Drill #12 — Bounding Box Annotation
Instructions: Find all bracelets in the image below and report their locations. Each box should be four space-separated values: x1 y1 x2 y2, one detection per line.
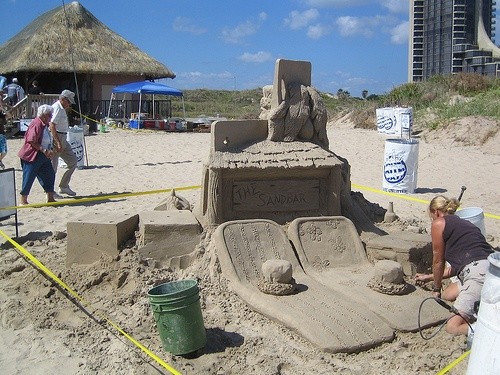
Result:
433 287 441 292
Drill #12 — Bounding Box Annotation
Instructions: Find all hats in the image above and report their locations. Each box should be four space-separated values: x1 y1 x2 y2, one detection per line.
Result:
61 89 76 105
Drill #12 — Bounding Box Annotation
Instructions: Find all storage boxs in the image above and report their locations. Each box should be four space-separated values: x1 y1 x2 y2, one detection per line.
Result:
130 119 144 129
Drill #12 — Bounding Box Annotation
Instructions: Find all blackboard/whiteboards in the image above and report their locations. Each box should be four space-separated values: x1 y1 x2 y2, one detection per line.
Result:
0 168 17 218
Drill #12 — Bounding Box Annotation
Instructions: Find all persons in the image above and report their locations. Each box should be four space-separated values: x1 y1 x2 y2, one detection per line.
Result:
415 195 495 334
28 80 44 119
18 104 63 208
0 109 8 169
43 89 78 199
3 78 21 121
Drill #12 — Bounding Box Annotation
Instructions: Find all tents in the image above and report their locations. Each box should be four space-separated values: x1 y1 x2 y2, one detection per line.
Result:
107 81 187 132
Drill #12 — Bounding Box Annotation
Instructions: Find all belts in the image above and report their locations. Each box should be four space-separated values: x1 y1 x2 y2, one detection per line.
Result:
56 131 67 134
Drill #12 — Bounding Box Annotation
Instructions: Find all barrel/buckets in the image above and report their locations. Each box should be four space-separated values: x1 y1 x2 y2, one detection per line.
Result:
454 207 486 239
382 138 420 195
147 280 207 355
58 126 85 171
466 251 500 375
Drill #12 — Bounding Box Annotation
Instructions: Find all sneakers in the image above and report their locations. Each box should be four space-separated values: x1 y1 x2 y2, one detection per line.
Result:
59 188 76 196
46 191 63 199
0 161 5 168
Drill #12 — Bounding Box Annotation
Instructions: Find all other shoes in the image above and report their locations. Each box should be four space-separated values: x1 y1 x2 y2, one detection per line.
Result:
18 203 36 208
46 201 61 206
